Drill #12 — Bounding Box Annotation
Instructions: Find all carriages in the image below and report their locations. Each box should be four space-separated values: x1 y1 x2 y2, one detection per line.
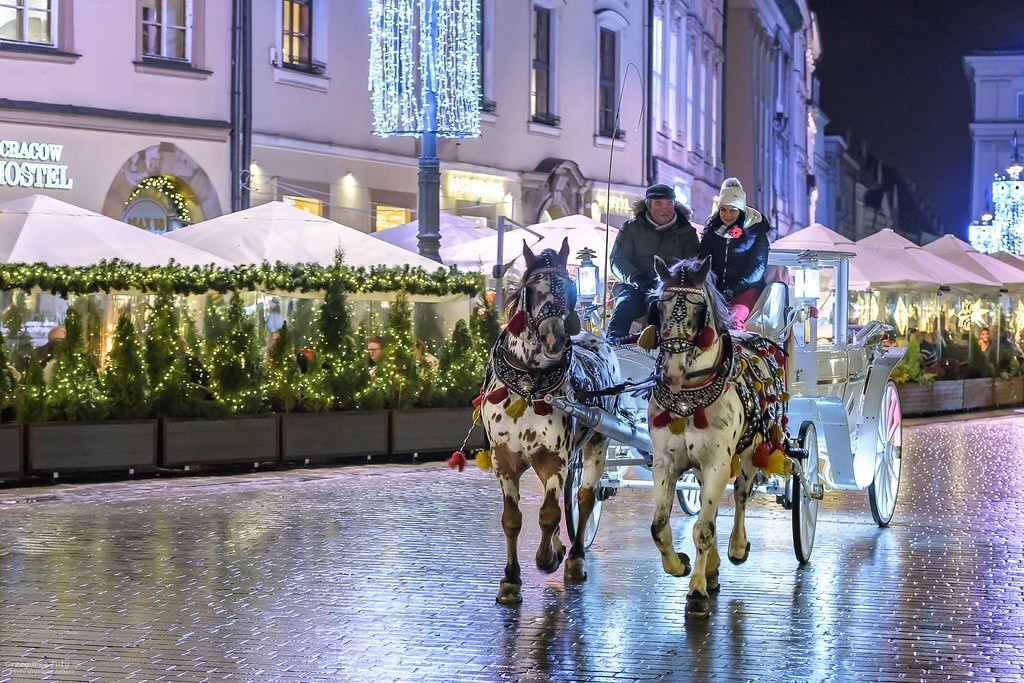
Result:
478 235 908 618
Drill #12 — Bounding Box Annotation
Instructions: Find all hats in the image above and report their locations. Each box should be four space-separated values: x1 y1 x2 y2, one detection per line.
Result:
645 183 676 199
718 177 746 213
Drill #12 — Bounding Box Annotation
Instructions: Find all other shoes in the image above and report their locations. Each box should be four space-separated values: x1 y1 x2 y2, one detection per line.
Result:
637 325 658 349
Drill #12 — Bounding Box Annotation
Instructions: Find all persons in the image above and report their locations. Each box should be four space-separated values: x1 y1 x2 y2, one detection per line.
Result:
698 177 770 334
605 183 701 351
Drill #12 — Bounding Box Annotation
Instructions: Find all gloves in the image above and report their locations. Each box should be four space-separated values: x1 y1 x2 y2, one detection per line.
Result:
721 286 734 300
631 274 654 293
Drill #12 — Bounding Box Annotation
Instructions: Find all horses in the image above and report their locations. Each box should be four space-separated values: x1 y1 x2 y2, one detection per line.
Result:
647 255 792 605
482 237 621 605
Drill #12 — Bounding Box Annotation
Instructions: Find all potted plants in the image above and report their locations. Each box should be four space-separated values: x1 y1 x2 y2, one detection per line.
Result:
891 332 1024 415
1 250 502 486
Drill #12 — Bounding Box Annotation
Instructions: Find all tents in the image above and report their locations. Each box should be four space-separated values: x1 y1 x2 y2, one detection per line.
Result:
3 195 1024 344
